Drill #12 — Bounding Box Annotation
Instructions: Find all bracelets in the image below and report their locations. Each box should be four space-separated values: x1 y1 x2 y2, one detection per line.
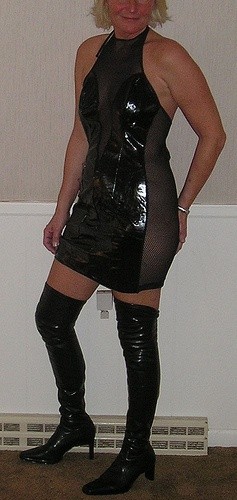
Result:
176 205 191 215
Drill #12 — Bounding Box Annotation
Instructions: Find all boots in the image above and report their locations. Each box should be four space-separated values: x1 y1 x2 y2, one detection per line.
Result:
17 282 97 463
82 296 161 496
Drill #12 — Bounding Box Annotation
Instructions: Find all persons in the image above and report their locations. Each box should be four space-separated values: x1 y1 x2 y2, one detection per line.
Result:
18 0 225 494
